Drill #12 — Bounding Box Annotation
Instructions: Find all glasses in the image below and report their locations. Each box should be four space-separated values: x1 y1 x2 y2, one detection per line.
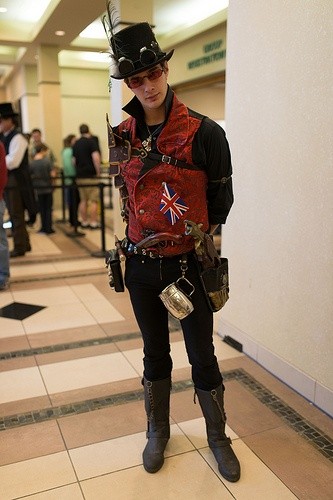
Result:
118 46 157 75
124 63 165 89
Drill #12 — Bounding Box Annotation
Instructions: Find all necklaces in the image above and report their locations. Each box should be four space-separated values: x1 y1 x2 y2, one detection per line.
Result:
143 122 165 151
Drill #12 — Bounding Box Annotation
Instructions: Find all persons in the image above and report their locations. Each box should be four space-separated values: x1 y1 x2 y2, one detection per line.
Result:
105 22 241 483
0 103 31 258
0 141 11 291
72 124 102 229
0 128 55 237
61 134 83 226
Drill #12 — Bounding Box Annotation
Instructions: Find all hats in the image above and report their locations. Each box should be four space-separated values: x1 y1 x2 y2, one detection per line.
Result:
100 0 175 79
0 103 19 119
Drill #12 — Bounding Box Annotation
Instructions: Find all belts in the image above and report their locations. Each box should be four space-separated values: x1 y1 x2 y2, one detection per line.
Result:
125 242 163 259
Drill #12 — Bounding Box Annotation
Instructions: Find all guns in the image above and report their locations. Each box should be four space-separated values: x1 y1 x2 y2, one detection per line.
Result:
184 219 221 271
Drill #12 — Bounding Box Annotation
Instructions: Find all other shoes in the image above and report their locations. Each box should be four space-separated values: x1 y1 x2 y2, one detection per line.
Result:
0 278 10 290
9 250 24 256
24 245 31 251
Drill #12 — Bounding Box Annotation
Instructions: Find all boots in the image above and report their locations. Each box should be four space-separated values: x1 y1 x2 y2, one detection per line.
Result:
142 373 171 474
193 378 240 483
